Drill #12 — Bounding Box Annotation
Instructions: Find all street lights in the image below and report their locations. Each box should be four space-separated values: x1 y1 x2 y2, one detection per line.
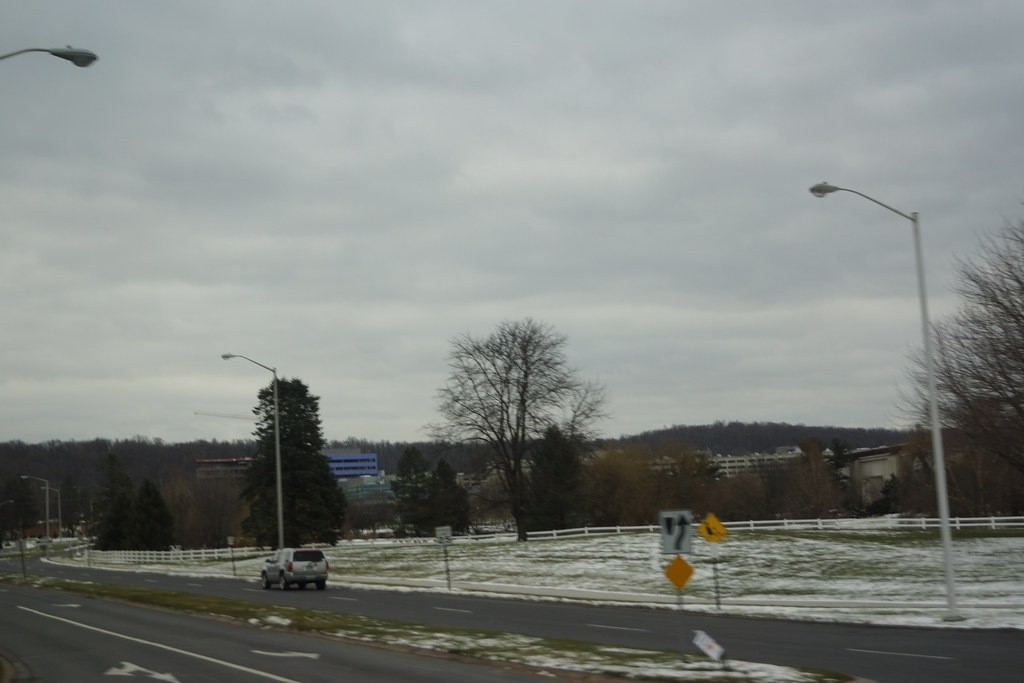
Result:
808 181 969 621
39 486 63 538
20 475 50 539
221 353 285 549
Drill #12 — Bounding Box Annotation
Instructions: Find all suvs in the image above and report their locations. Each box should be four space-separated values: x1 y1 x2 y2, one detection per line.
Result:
260 548 330 592
